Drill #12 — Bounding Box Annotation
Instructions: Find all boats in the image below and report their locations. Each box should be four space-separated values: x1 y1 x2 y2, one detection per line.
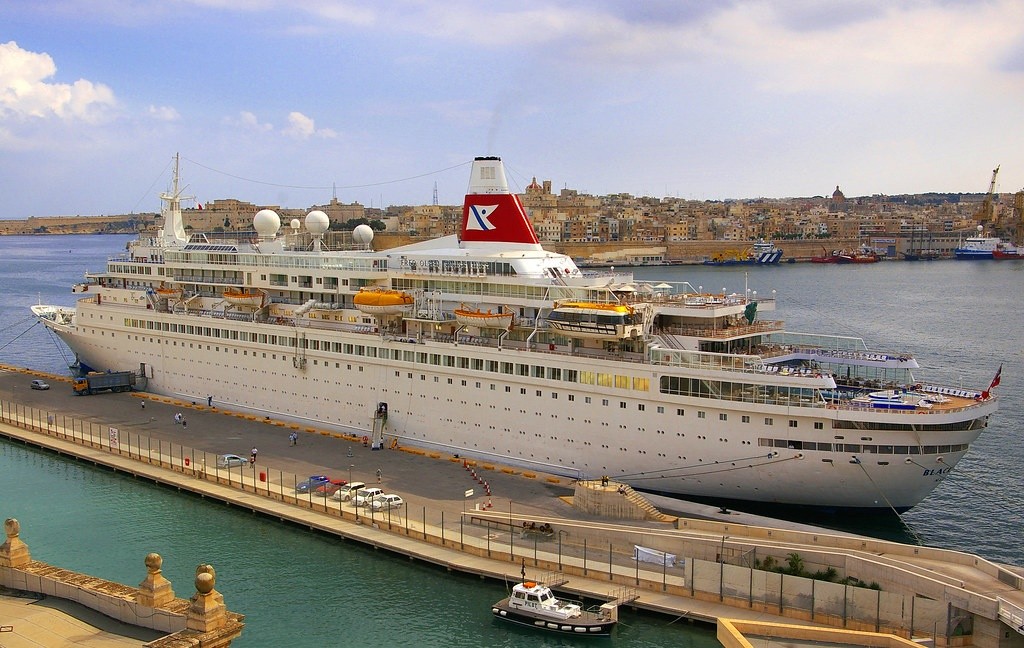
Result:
841 243 874 263
955 232 1017 259
155 288 182 298
545 302 644 339
634 544 676 564
353 291 414 313
704 237 784 265
453 309 512 328
491 557 617 637
223 291 267 304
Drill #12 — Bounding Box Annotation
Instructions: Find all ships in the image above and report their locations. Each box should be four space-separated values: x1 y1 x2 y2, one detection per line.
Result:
30 151 998 521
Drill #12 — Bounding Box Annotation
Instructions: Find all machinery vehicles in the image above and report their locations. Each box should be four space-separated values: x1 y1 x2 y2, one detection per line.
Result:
713 247 733 262
735 247 754 261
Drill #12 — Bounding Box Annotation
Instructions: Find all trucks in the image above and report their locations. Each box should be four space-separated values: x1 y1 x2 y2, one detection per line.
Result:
72 369 136 396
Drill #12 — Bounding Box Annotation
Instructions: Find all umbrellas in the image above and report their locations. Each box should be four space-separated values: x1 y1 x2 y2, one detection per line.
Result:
617 281 674 299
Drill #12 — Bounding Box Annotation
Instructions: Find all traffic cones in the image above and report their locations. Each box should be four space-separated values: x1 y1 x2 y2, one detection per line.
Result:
466 463 471 470
486 497 493 507
463 460 467 468
478 475 484 484
470 468 474 475
472 471 478 480
483 480 487 487
481 502 487 511
485 484 491 492
486 488 491 496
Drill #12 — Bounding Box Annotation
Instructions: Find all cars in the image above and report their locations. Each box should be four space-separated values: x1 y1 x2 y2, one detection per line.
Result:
368 494 403 511
296 476 330 493
334 482 366 501
217 454 247 468
352 488 384 507
316 479 347 496
31 380 49 389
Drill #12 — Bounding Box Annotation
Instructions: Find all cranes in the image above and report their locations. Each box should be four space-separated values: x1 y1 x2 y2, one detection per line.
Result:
973 164 1000 224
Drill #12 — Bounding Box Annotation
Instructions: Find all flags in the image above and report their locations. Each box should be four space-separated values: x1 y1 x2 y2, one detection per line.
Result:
198 204 202 210
991 365 1001 388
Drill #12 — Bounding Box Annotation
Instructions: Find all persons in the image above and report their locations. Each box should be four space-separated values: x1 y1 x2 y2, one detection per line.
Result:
618 485 627 495
289 432 297 446
379 437 383 449
249 455 254 468
174 410 186 430
376 469 382 484
207 396 212 406
141 400 145 408
601 474 608 486
378 404 386 418
252 447 257 461
